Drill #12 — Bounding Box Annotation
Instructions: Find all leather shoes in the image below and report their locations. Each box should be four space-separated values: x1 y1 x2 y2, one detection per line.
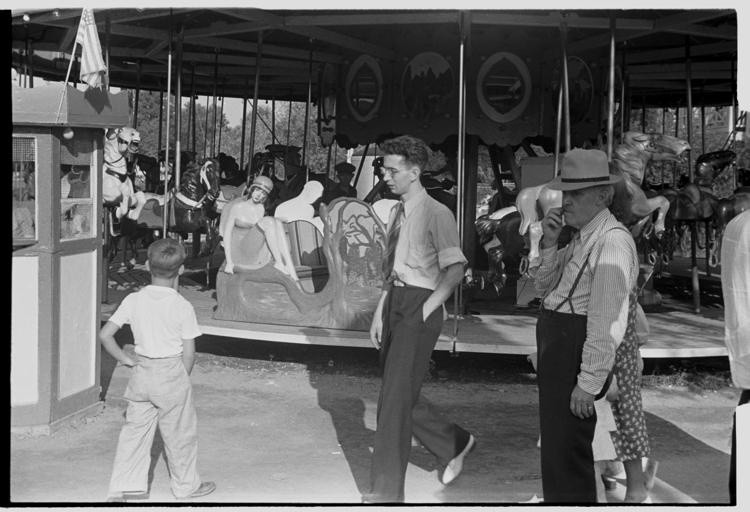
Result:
441 433 476 486
185 481 218 498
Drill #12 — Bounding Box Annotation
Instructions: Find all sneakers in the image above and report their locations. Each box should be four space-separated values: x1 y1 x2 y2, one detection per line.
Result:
642 458 660 491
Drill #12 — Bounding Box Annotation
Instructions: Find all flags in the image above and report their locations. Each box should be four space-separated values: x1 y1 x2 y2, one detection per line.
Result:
75 8 107 90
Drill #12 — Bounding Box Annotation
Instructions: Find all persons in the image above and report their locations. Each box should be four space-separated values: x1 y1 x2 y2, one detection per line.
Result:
364 135 475 503
605 285 659 505
97 237 216 504
529 148 641 502
224 159 400 281
719 209 749 505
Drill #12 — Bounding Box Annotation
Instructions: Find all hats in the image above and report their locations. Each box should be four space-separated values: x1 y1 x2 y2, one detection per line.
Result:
546 148 622 193
